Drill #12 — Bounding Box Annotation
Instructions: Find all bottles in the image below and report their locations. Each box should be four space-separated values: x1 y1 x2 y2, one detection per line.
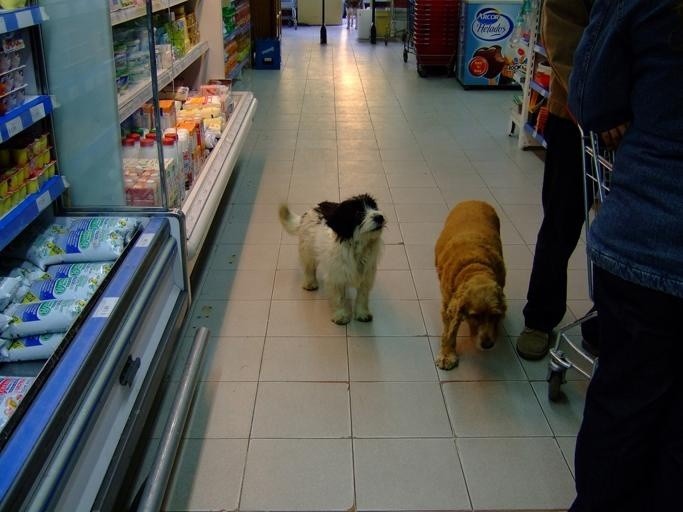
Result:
121 131 185 202
223 2 251 78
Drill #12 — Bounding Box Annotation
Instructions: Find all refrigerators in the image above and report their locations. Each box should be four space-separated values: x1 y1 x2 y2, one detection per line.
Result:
455 0 536 89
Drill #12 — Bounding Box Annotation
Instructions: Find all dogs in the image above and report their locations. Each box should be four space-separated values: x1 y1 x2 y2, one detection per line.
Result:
278 192 388 326
434 199 507 371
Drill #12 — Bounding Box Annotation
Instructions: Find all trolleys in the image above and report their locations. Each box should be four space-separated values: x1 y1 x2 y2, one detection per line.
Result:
547 123 614 401
384 7 413 62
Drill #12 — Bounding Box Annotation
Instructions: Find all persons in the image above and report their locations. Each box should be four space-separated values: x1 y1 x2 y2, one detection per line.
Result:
568 1 682 509
517 1 624 359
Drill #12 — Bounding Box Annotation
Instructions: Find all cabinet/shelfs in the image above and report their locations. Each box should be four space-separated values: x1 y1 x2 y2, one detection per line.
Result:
35 0 258 300
2 0 194 512
508 0 549 151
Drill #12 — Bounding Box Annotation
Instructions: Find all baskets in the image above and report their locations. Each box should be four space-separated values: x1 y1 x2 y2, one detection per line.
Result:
414 0 459 77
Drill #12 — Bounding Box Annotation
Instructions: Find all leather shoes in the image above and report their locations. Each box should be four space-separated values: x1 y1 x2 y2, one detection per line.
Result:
517 327 549 360
